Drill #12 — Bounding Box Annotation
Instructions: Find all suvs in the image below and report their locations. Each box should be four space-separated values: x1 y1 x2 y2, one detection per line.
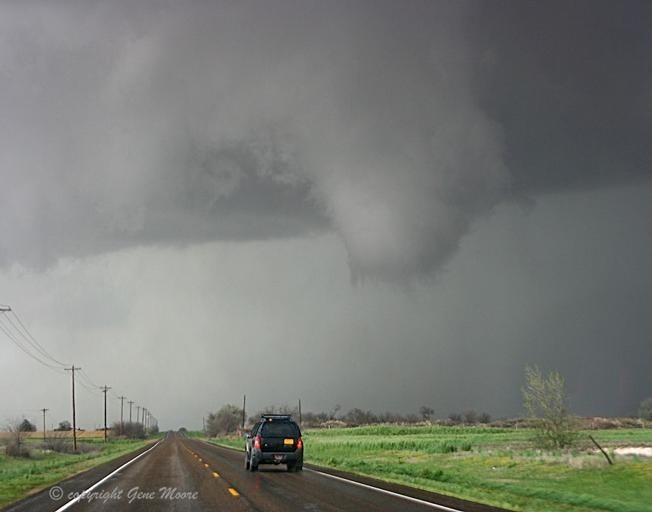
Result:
243 411 305 474
